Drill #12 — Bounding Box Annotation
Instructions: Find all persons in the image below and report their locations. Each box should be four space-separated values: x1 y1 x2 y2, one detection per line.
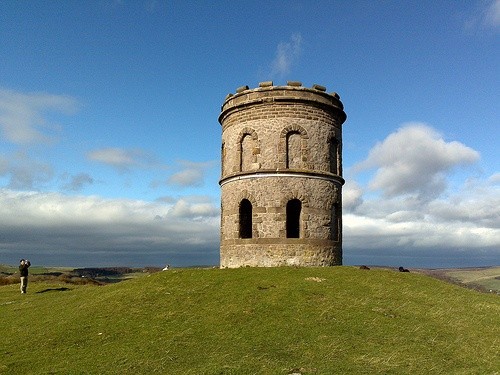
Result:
18 259 32 294
